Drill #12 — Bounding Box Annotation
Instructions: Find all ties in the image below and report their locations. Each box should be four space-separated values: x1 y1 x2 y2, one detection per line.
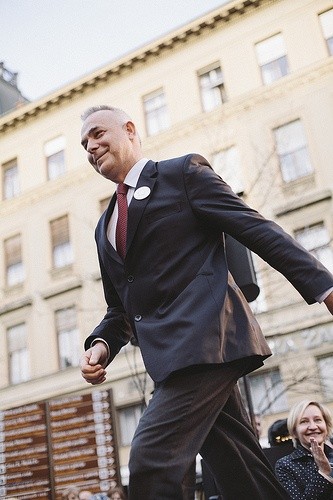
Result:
115 181 129 259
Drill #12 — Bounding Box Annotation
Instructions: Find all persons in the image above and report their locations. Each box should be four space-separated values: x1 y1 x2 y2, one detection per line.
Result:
248 399 333 500
78 105 332 500
